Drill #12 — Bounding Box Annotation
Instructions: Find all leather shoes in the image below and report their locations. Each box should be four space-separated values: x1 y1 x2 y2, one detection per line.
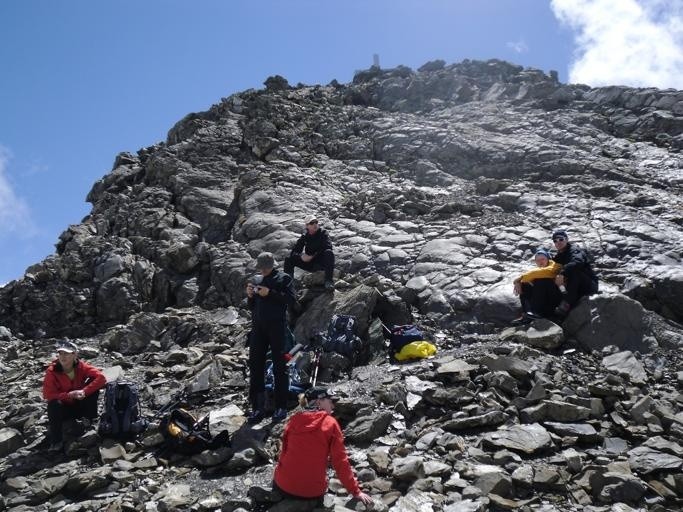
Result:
272 407 286 420
248 408 266 423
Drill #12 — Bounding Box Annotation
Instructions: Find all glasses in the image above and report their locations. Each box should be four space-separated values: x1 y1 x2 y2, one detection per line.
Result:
59 352 73 356
552 237 565 243
309 220 318 225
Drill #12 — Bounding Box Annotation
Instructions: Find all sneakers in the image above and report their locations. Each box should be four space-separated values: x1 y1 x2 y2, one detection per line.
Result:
48 442 64 453
221 498 255 512
325 282 335 292
509 300 570 326
248 485 282 503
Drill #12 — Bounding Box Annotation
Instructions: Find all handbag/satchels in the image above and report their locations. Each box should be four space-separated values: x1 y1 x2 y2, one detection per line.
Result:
160 408 230 454
389 324 425 353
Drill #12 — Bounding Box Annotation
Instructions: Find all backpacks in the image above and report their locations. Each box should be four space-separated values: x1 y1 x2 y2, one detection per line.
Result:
327 313 358 343
98 378 148 437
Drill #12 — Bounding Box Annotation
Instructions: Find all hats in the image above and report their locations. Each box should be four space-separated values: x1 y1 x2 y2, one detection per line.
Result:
57 342 80 353
536 249 549 258
553 230 567 240
304 387 340 403
254 252 279 270
305 214 317 224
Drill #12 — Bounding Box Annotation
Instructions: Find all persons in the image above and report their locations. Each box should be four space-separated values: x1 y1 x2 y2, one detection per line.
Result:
283 213 335 295
510 247 565 318
246 248 295 422
42 340 103 438
246 386 372 506
549 231 601 309
